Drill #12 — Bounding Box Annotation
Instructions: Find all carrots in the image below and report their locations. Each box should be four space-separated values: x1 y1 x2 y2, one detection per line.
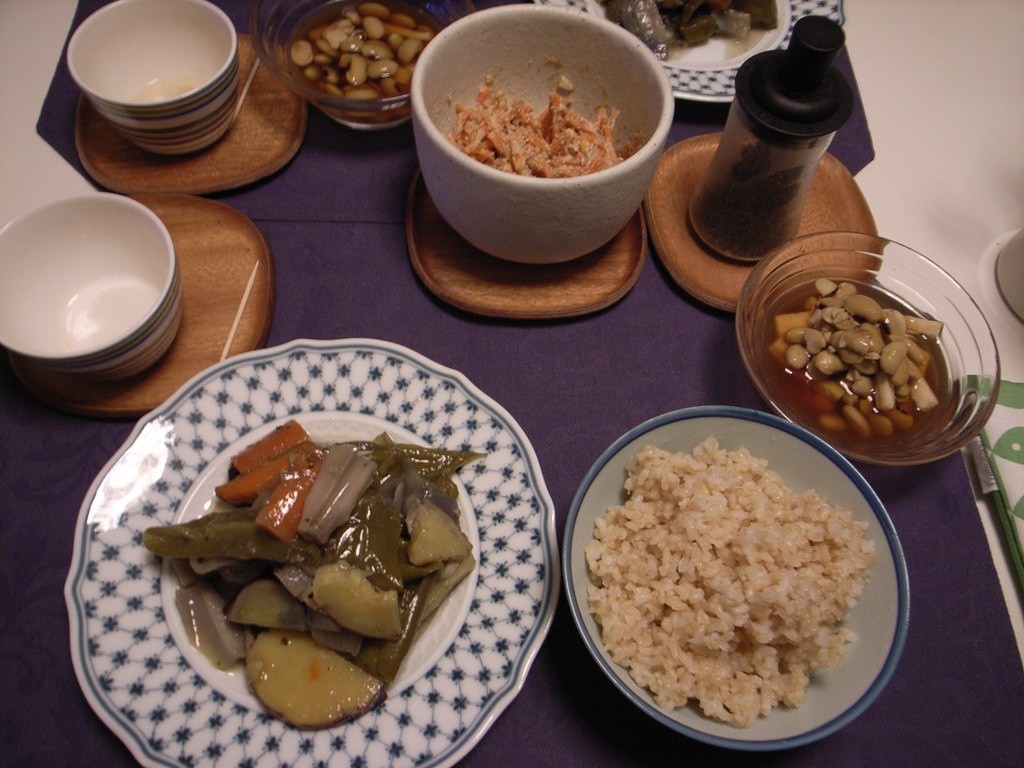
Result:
216 419 329 543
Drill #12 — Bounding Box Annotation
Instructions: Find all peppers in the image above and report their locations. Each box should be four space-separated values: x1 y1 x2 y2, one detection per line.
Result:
142 432 493 683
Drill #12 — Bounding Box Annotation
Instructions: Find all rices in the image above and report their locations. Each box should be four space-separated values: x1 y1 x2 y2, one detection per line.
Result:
584 434 875 729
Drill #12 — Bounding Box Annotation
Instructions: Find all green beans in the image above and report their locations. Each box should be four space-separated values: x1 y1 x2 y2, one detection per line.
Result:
304 2 436 99
601 1 777 45
781 278 926 440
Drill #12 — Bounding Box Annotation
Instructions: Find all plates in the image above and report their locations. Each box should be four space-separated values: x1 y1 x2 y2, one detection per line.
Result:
531 0 845 102
74 32 307 193
644 130 882 311
63 336 560 768
18 191 274 414
405 163 648 319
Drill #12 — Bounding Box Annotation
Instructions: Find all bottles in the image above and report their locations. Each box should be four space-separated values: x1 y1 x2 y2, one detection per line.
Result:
689 15 855 265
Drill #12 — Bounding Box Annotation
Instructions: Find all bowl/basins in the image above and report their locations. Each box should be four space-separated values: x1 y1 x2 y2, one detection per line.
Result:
735 230 1002 465
66 0 240 158
410 4 674 265
562 405 912 751
0 192 183 381
248 0 477 132
